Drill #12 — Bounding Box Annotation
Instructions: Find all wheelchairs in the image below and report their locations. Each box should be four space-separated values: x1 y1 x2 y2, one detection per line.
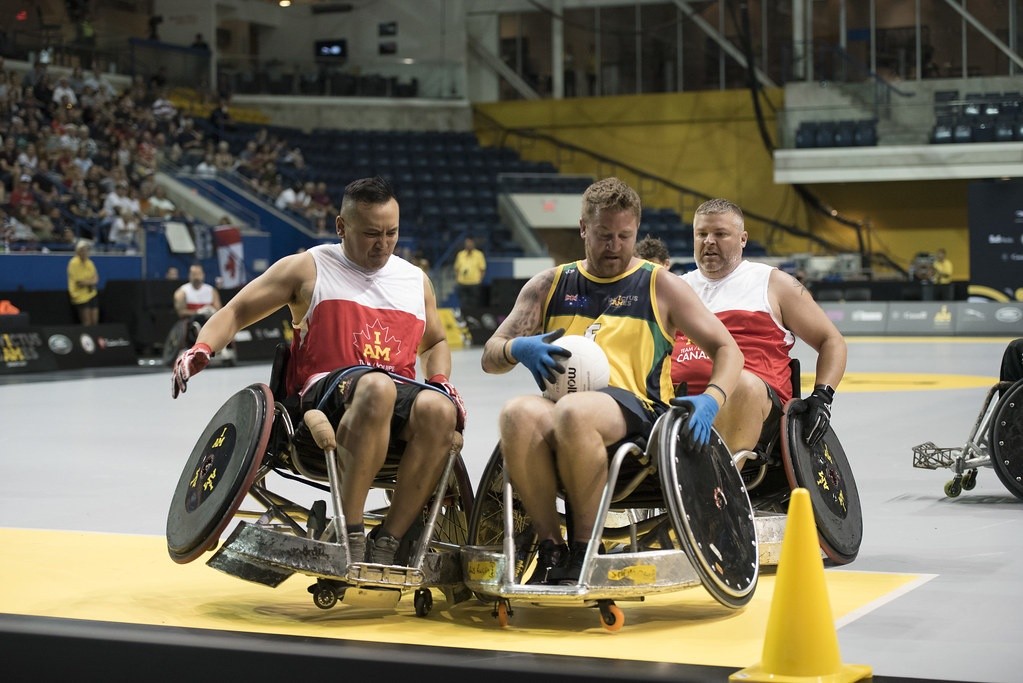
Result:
911 338 1023 503
165 342 476 618
626 359 864 566
462 381 760 631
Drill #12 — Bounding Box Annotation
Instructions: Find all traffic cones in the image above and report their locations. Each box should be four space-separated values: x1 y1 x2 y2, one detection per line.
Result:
727 486 874 682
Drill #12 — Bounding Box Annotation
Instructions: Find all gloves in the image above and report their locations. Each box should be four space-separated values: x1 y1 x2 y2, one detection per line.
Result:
668 394 719 453
170 343 211 400
427 374 465 432
512 327 572 391
792 383 835 448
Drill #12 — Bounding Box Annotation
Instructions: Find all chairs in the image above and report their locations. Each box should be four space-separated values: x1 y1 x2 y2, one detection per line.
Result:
208 125 697 272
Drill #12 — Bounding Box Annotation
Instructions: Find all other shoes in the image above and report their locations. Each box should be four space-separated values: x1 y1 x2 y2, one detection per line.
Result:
347 533 366 562
552 541 607 588
520 539 569 585
365 532 400 568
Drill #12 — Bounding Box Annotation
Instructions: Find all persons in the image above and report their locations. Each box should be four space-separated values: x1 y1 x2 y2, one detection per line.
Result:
170 178 464 565
0 32 433 359
999 339 1023 396
931 248 954 300
482 177 745 585
672 199 848 474
633 234 671 271
453 235 487 318
